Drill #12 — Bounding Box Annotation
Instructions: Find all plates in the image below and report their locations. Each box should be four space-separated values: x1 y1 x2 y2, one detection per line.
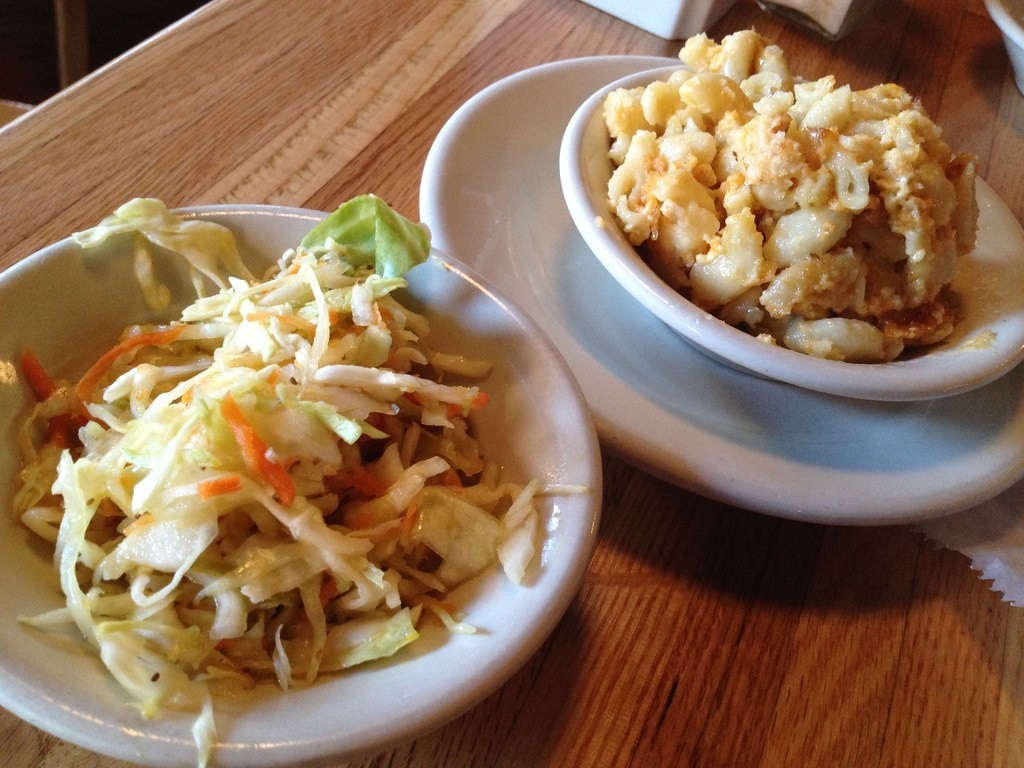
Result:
423 52 1024 522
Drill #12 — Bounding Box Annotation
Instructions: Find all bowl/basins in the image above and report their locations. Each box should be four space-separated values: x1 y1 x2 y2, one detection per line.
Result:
559 64 1024 405
0 201 604 768
983 2 1024 98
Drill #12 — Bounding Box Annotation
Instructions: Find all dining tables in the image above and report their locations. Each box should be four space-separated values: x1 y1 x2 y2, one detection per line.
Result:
0 0 1023 768
